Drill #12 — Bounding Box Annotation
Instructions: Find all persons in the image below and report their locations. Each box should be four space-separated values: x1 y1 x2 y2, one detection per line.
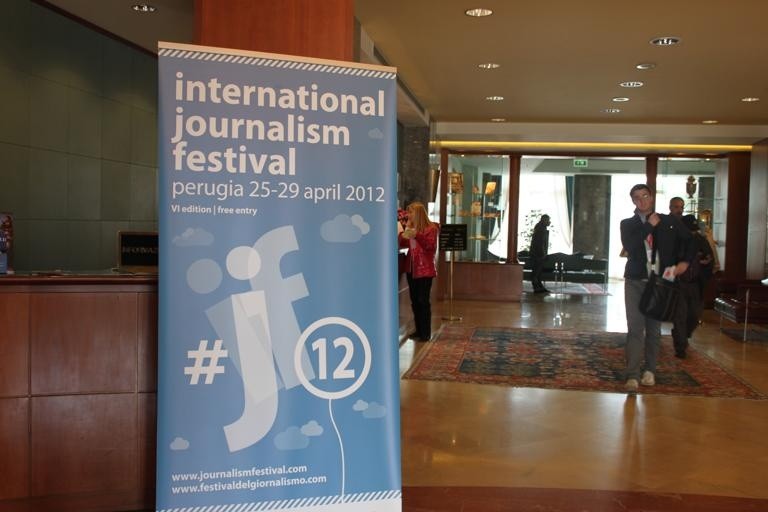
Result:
530 214 550 293
620 184 697 391
398 202 437 342
672 214 714 359
667 197 684 219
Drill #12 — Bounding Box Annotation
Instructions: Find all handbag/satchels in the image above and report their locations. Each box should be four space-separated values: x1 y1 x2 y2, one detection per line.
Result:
638 273 689 323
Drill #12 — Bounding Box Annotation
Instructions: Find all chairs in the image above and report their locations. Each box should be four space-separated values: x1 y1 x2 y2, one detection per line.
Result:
484 246 612 300
708 274 767 344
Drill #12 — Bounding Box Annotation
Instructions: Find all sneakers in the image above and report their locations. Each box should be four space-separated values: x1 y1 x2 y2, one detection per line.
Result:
672 328 688 358
624 377 639 392
534 287 551 293
408 330 430 342
640 371 655 385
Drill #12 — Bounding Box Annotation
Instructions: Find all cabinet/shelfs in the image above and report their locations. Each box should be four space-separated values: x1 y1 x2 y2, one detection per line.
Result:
682 195 727 250
444 191 507 248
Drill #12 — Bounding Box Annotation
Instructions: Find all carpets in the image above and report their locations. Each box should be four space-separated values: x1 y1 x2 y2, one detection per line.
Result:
399 321 767 403
